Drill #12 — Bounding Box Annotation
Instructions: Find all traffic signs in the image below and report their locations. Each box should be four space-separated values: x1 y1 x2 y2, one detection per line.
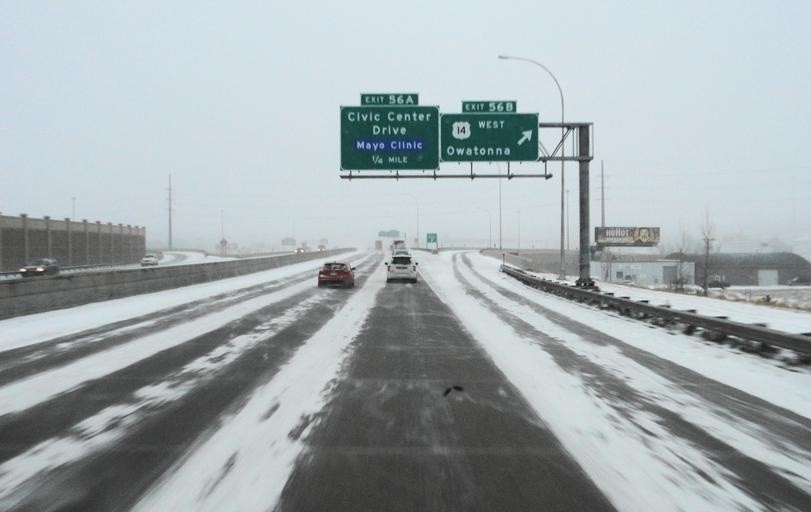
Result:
379 229 401 238
463 102 518 113
363 93 419 106
342 104 438 169
441 112 539 162
427 233 438 242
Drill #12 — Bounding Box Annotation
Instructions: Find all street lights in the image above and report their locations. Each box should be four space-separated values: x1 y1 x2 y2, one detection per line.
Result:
498 54 569 279
72 195 78 221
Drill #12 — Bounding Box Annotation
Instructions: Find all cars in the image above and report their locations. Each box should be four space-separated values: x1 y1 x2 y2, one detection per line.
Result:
142 253 159 265
702 280 729 288
318 245 327 251
788 276 810 286
672 277 690 285
295 247 305 255
386 239 418 282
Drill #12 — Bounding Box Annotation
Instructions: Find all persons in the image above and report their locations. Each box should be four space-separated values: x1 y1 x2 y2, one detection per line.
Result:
633 227 657 245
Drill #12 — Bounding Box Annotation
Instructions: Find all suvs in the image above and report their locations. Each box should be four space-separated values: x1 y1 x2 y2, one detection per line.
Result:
318 259 357 288
20 258 60 277
